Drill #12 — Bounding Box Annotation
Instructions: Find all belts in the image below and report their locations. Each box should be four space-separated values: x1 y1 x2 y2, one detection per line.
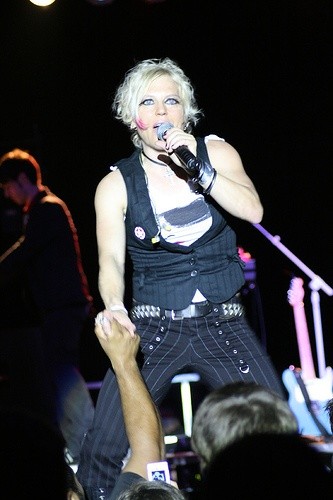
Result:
131 303 244 320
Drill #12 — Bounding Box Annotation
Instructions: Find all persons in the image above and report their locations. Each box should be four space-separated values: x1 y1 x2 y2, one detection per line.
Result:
0 309 333 500
76 56 286 500
0 148 96 405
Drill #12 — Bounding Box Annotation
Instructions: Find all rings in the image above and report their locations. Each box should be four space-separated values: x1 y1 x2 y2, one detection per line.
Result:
98 316 107 323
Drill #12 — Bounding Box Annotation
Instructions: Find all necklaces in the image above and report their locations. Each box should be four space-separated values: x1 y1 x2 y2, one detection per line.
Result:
139 148 177 176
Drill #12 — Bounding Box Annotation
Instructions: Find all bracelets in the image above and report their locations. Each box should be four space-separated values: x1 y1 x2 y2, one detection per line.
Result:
192 158 217 195
110 305 128 314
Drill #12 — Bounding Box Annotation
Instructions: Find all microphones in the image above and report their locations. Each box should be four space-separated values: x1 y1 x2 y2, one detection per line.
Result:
157 122 202 171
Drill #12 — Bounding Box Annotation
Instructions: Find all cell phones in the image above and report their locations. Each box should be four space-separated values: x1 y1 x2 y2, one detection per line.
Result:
147 461 170 484
